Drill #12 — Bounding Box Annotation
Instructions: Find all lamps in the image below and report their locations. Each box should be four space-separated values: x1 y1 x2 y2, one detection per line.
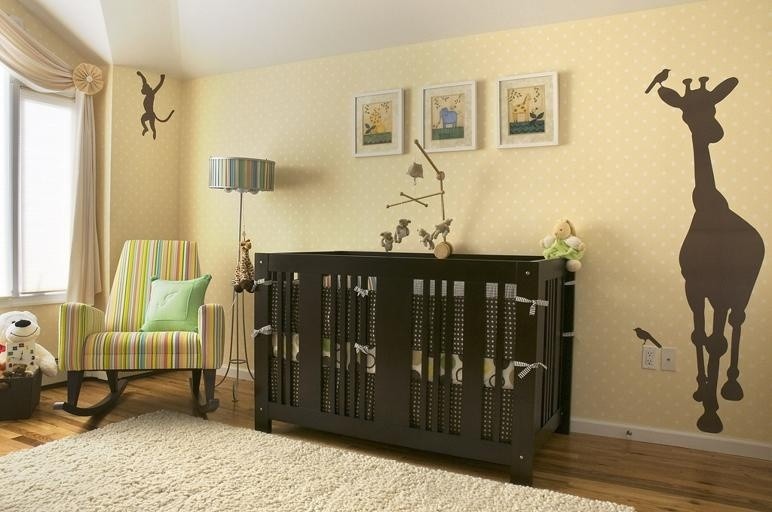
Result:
207 156 276 401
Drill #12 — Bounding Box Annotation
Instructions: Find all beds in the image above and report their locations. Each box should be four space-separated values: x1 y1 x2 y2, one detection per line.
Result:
249 248 579 488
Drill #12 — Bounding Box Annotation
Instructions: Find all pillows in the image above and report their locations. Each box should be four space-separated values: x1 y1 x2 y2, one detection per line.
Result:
135 271 214 332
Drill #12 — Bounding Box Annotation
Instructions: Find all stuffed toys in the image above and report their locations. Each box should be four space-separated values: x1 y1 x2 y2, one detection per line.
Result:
0 311 58 388
539 222 585 272
233 238 255 293
380 138 454 259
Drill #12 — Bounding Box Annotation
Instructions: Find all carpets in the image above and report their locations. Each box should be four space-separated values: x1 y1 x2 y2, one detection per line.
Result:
2 406 639 511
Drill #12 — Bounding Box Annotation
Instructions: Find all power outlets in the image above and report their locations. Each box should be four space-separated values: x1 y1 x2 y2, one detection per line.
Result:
641 345 658 370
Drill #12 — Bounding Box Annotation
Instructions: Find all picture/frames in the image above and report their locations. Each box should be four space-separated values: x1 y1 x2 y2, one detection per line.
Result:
352 87 406 159
497 71 560 150
420 81 478 153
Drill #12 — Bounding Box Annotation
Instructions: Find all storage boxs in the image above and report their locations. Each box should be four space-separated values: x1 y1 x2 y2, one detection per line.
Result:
0 373 43 422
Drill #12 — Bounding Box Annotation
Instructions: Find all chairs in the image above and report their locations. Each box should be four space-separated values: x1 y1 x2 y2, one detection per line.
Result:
53 234 227 420
